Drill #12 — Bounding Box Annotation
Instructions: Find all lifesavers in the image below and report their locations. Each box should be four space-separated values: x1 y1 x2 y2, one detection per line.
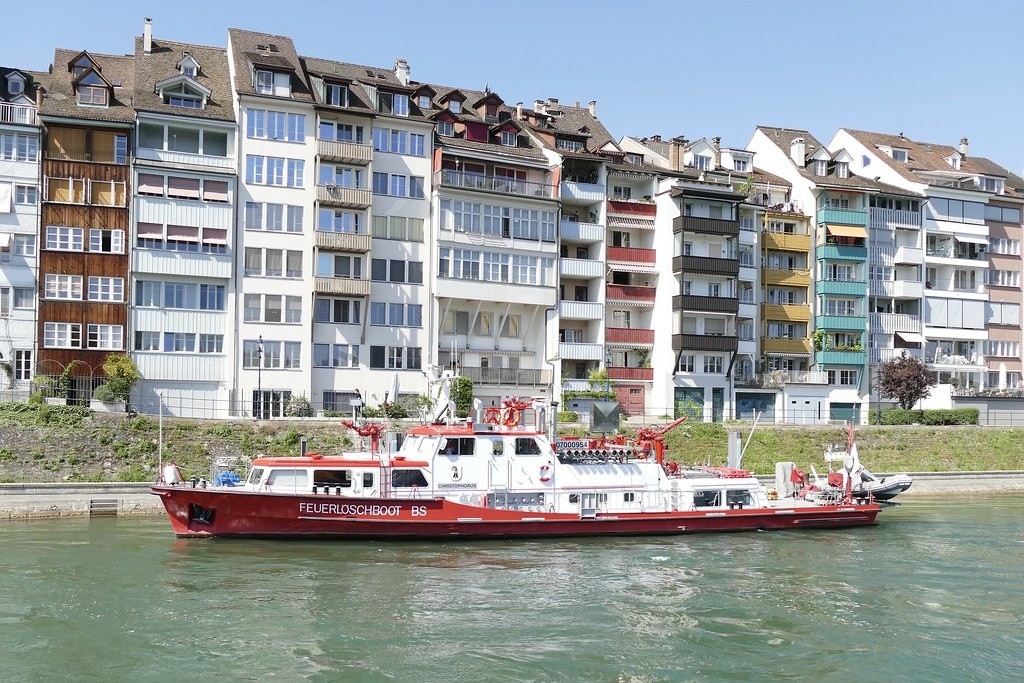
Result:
501 406 522 428
632 440 651 460
483 406 502 426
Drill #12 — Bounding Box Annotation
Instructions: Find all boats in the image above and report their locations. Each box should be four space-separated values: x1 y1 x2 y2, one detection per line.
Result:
810 400 913 501
148 396 882 542
